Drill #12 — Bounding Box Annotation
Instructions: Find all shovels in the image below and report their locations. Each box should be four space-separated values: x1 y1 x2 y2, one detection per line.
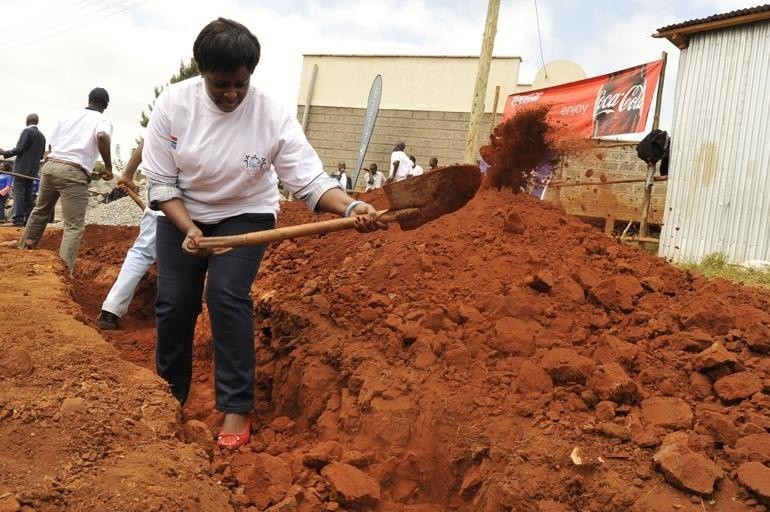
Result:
188 164 481 250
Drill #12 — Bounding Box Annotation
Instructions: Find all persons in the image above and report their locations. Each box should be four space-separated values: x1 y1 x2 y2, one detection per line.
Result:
329 141 438 196
142 17 389 448
17 88 112 279
95 136 157 329
0 114 46 226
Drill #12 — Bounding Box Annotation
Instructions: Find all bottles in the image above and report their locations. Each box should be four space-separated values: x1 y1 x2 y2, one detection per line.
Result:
590 70 624 138
624 64 649 134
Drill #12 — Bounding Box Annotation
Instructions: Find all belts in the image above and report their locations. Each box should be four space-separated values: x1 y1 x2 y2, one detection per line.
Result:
47 157 87 175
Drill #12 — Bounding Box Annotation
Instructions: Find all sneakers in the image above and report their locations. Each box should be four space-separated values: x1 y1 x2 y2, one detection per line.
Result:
95 310 118 329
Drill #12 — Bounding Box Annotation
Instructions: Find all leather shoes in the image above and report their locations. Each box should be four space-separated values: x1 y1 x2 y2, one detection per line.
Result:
12 216 24 225
217 415 251 449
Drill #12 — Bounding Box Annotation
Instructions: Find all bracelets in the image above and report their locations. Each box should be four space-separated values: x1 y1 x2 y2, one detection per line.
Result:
345 201 362 217
